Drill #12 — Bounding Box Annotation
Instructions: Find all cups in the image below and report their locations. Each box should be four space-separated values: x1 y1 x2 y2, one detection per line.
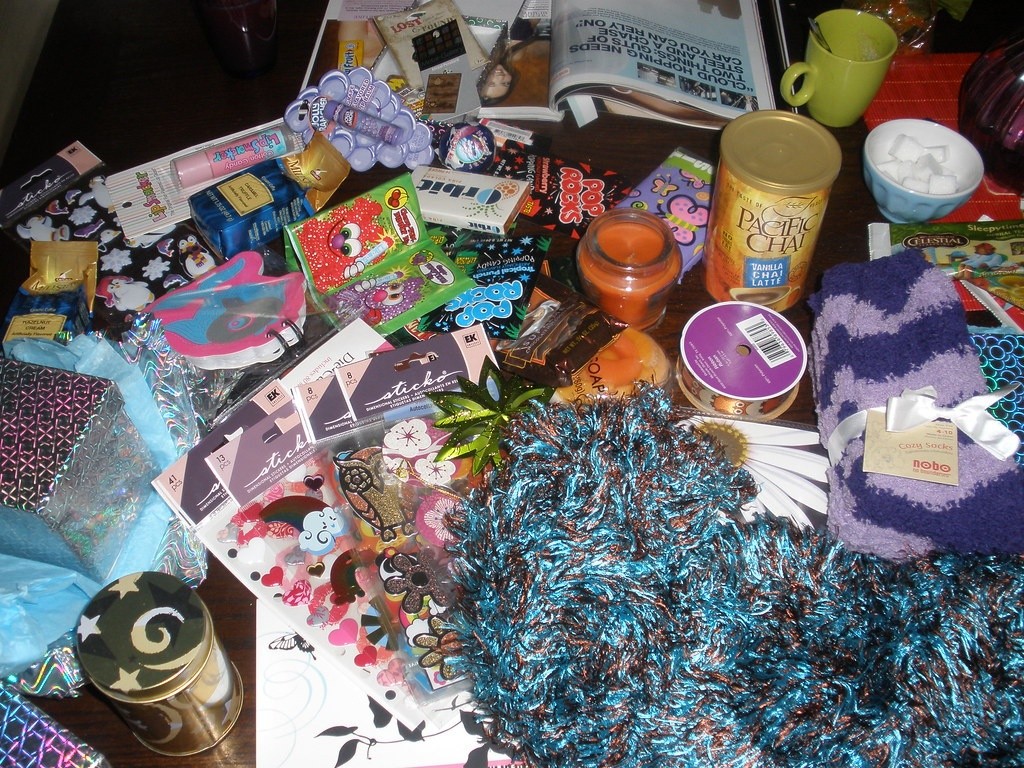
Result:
779 9 898 128
74 571 245 758
193 0 278 79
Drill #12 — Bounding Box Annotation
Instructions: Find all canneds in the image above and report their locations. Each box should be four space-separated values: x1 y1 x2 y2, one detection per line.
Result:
700 112 842 314
575 208 682 329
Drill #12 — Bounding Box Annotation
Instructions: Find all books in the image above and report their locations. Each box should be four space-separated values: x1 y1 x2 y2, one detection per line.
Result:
297 0 777 134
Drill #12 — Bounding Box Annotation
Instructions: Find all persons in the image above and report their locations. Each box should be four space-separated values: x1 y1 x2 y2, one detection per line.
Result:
476 18 544 105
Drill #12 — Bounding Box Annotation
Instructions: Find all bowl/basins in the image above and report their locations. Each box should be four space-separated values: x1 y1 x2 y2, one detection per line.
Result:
863 118 985 225
